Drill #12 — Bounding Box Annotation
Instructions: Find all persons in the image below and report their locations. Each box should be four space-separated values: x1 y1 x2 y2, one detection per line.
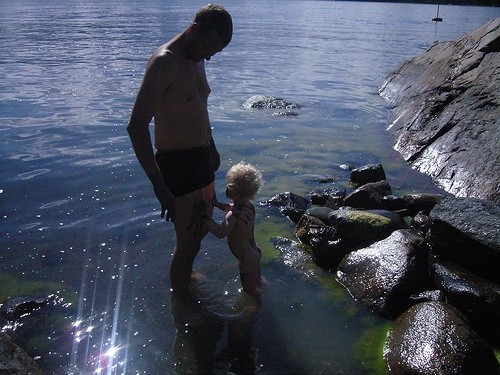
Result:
202 161 263 293
127 3 232 284
